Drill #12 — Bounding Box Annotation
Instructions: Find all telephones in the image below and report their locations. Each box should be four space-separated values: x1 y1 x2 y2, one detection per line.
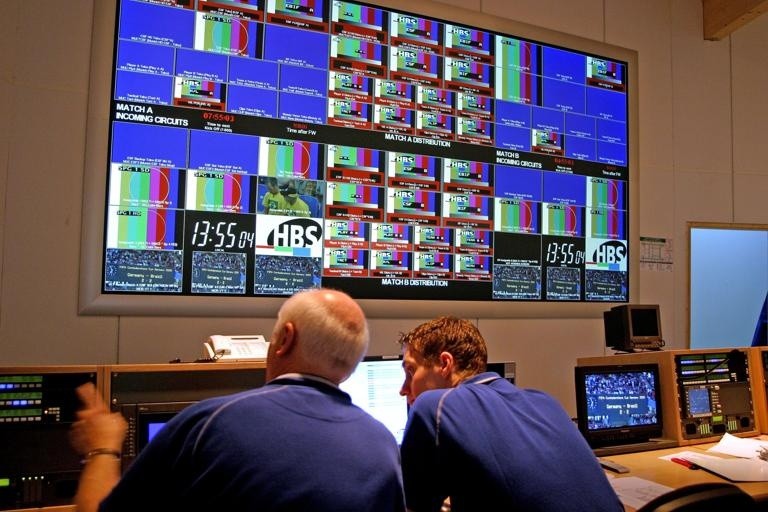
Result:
202 334 268 362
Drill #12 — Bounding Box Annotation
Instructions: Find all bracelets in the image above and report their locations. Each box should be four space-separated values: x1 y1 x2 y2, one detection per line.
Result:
85 447 122 460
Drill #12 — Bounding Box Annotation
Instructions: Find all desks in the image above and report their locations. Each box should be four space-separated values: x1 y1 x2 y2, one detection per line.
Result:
594 434 768 510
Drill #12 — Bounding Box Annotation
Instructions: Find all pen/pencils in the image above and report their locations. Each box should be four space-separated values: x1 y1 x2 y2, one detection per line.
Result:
671 457 699 470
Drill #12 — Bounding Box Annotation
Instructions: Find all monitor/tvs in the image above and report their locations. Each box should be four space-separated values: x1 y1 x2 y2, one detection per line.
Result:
134 412 182 455
574 363 664 450
602 304 664 350
338 355 409 446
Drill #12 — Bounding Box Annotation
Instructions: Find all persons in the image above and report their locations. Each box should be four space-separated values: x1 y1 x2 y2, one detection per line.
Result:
395 314 627 512
585 372 657 430
260 177 317 217
66 287 411 512
